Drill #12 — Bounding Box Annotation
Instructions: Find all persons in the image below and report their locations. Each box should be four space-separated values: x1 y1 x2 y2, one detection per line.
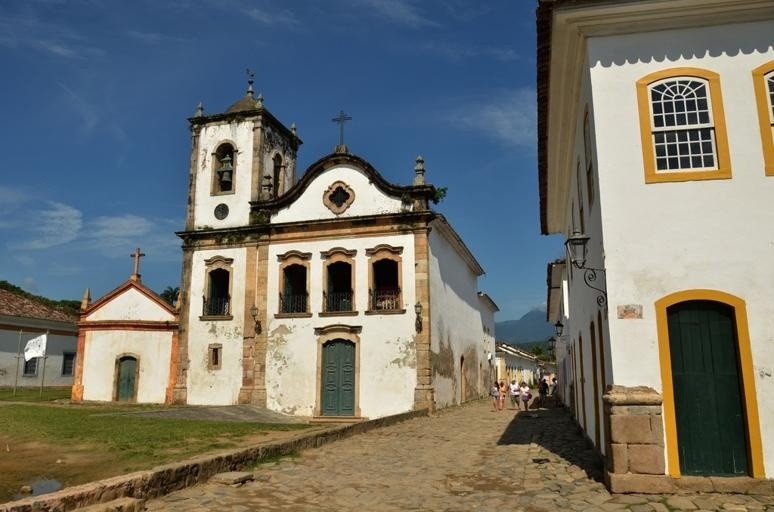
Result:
490 379 530 413
537 378 562 408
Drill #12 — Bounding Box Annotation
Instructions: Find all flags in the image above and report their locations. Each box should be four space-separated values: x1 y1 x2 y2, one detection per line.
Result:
23 333 47 362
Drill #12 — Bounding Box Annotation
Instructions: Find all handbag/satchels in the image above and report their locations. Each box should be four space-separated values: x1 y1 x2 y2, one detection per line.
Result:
528 393 532 399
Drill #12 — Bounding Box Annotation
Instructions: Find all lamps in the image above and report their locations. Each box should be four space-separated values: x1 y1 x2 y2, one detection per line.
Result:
250 306 262 333
566 227 607 308
548 319 570 353
414 302 425 332
